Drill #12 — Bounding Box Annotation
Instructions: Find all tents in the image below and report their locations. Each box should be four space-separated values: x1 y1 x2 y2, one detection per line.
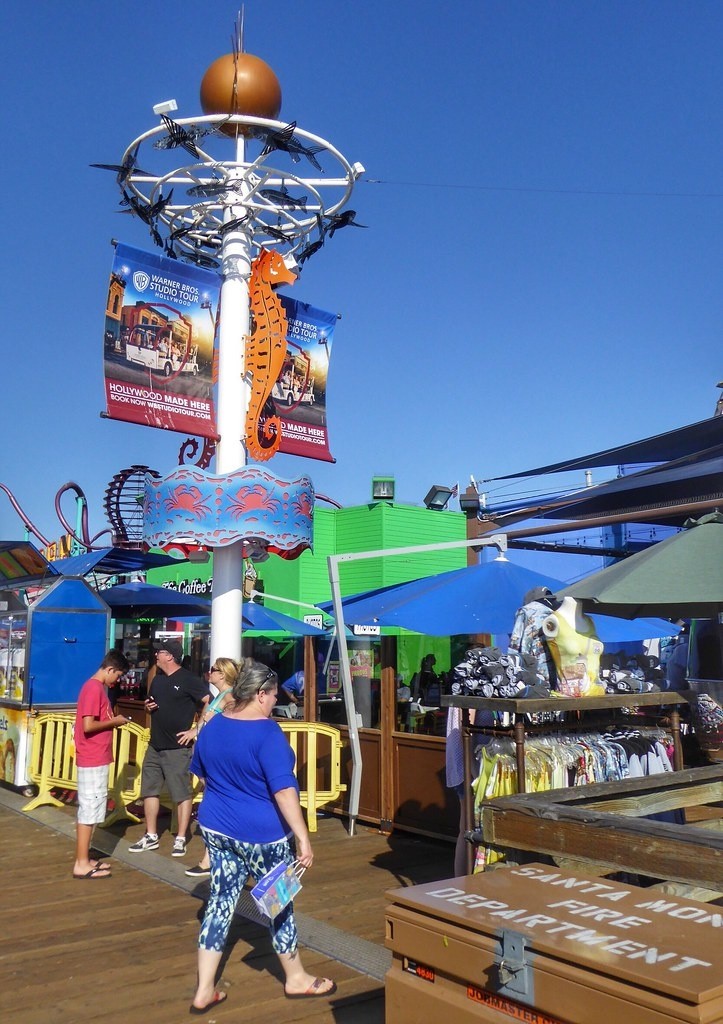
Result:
467 414 723 647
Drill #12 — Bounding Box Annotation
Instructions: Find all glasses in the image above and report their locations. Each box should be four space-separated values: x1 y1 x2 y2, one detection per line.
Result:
155 650 169 655
258 666 277 695
210 667 220 674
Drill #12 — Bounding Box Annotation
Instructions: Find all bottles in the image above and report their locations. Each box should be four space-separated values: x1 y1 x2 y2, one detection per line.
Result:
116 671 136 696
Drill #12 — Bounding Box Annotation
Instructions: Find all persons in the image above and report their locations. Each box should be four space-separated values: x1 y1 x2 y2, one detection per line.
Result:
182 619 689 740
191 657 337 1014
277 370 310 395
186 658 240 876
447 641 495 880
129 638 215 857
544 597 604 697
146 337 194 363
72 648 132 878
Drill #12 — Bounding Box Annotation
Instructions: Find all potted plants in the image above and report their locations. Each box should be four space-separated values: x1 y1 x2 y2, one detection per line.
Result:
699 739 721 762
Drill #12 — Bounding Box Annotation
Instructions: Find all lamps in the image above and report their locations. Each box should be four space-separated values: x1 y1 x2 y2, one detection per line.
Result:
371 476 395 502
459 494 480 512
423 484 453 510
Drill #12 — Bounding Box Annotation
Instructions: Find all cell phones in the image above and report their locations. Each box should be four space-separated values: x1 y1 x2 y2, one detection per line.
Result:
126 717 132 719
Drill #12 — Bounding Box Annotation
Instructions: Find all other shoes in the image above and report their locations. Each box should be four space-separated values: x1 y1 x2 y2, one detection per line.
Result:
184 862 211 876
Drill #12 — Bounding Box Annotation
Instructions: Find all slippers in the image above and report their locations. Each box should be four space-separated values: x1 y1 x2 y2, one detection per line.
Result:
189 991 228 1014
95 862 111 870
284 976 336 999
72 868 112 879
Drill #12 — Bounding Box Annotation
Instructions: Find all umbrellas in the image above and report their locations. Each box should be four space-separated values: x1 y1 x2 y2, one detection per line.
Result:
167 601 327 635
313 560 683 645
553 505 723 618
97 581 255 626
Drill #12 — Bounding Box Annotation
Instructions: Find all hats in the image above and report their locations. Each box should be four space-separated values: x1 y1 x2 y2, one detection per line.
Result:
525 587 556 605
153 639 183 660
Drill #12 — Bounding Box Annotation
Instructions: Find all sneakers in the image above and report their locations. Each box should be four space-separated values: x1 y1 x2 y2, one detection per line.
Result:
128 835 161 852
171 839 186 857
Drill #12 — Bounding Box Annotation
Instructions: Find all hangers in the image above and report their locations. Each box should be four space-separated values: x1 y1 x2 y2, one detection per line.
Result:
474 718 674 777
